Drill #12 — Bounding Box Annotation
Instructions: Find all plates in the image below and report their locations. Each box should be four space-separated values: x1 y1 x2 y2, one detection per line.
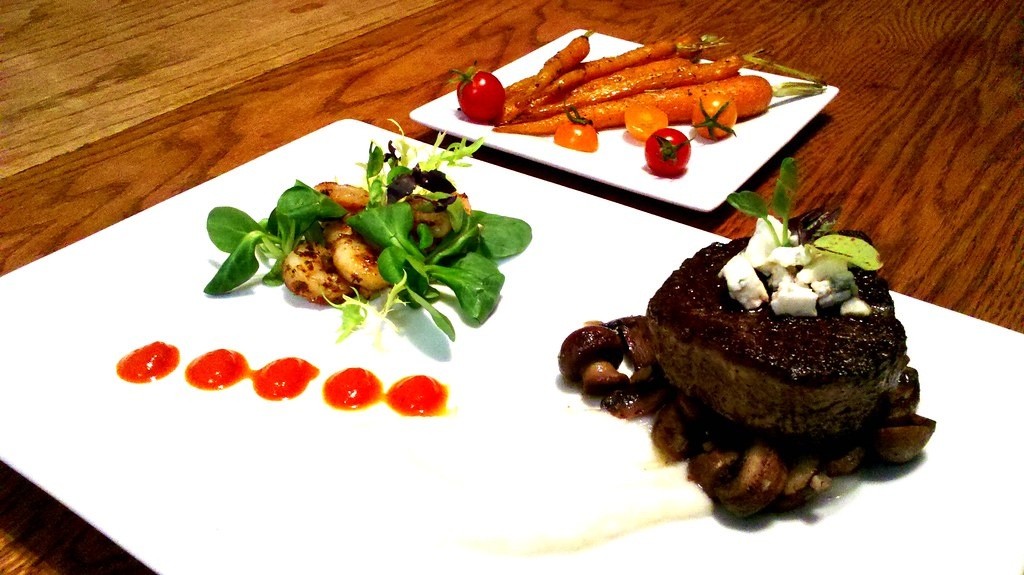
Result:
0 118 1024 575
408 28 839 213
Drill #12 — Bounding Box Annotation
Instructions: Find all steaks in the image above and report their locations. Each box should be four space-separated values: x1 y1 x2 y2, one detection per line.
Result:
645 236 908 436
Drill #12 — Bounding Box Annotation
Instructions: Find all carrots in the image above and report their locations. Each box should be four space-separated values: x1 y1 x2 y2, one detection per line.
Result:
492 33 771 135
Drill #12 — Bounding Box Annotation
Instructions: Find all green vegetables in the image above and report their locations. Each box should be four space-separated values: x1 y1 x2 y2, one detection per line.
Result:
728 158 885 271
205 119 534 348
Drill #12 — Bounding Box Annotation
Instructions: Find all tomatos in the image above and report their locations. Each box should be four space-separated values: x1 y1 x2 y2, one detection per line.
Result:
553 95 738 175
447 63 505 122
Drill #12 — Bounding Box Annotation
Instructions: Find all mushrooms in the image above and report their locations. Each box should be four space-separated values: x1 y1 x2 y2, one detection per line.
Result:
558 315 937 519
